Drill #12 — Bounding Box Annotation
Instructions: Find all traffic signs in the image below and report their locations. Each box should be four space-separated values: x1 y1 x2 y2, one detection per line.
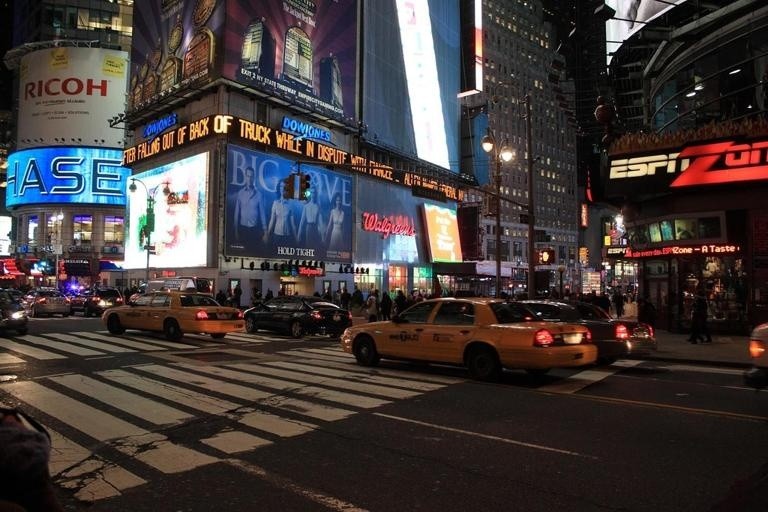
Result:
411 184 447 204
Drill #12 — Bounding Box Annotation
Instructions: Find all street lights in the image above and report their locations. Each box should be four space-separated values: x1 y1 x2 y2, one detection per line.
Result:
481 126 514 298
51 208 64 289
128 176 173 282
558 266 566 300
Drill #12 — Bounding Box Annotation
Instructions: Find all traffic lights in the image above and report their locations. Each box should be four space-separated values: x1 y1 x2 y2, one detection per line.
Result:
538 249 555 265
283 174 295 199
299 173 311 200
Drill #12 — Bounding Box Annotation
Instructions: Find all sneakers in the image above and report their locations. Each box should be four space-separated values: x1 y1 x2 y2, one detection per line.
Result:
703 339 713 343
700 337 704 343
691 341 698 345
686 339 691 342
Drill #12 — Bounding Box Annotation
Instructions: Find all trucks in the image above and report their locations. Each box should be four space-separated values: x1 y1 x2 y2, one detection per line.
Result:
137 276 195 294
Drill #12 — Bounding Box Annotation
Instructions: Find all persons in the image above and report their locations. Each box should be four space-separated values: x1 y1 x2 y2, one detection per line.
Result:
216 284 243 307
263 178 296 258
118 282 139 301
293 185 325 258
691 290 710 344
254 288 285 307
686 286 707 342
546 287 624 318
232 164 268 258
324 191 344 253
314 285 428 321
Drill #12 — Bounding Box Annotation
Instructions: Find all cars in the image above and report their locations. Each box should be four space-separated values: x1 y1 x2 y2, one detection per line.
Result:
102 280 246 340
743 323 768 393
242 295 352 338
0 284 124 333
